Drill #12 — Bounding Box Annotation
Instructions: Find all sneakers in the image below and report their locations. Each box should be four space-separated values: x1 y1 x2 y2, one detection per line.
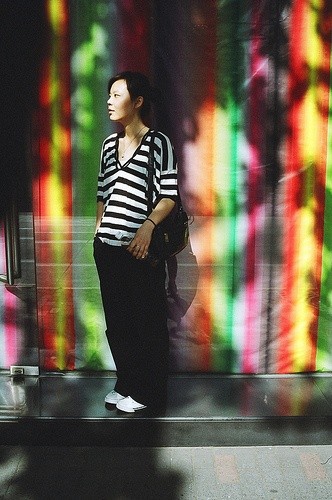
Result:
104 390 147 412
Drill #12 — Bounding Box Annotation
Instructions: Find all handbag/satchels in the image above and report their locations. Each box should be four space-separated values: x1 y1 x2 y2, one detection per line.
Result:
148 202 190 260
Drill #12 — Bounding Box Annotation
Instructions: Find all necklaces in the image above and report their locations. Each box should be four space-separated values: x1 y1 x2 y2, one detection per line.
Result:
122 125 146 158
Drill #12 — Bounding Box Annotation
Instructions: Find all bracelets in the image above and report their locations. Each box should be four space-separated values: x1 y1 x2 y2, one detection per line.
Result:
146 218 157 227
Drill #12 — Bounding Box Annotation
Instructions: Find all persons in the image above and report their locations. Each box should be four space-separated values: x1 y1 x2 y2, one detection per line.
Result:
93 71 178 413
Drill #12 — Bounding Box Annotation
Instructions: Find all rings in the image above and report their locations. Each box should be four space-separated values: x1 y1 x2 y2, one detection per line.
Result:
136 245 140 248
